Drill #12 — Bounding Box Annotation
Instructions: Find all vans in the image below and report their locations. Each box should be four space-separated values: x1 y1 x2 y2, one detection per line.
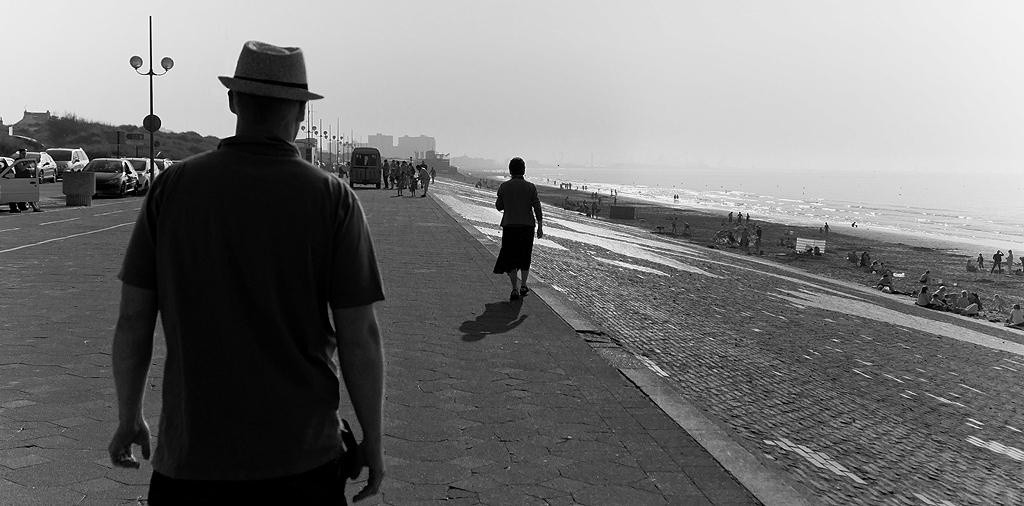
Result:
350 146 380 187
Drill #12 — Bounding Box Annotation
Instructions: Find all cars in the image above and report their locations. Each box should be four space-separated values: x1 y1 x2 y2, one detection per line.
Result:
1 146 185 205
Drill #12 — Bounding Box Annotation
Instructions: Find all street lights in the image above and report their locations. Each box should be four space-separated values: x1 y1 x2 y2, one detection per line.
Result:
302 125 355 165
130 54 174 186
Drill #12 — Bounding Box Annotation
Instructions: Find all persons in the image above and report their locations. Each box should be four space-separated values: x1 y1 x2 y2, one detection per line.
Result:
383 156 437 197
475 177 490 188
491 157 545 300
776 219 895 296
915 249 1022 328
654 194 763 257
109 38 386 504
14 148 42 212
548 179 618 219
338 165 348 178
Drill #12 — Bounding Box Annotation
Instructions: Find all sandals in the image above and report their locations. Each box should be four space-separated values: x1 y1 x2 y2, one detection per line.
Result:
521 285 529 294
511 288 519 299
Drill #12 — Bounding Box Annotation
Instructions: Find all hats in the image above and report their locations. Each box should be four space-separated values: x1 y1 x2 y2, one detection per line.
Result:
218 40 324 101
